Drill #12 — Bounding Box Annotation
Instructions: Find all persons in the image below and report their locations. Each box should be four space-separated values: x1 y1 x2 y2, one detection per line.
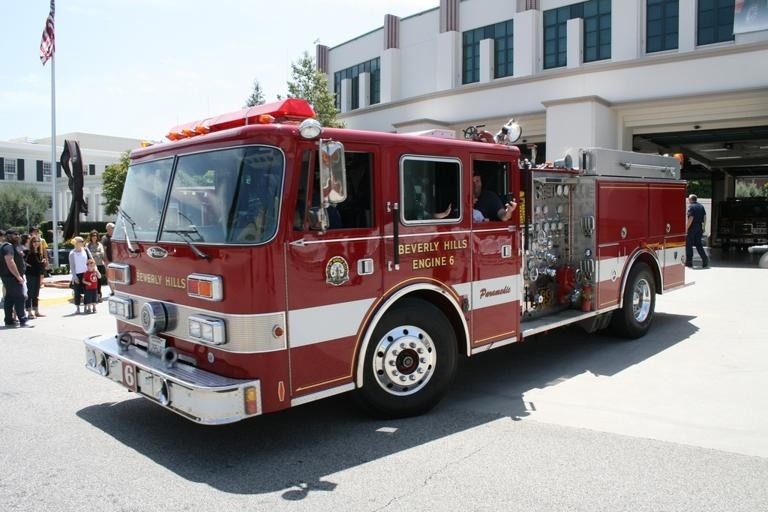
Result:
686 193 710 269
472 168 518 222
292 165 344 231
472 180 490 224
0 223 116 330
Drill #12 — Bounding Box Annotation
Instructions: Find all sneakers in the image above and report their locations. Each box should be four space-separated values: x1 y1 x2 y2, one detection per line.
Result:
28 313 45 319
75 309 96 314
5 321 35 328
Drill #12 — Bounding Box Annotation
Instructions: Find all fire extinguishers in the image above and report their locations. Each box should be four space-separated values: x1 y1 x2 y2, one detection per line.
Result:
582 282 592 312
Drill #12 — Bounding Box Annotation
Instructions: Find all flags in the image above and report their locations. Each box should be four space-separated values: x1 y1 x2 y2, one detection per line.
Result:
38 0 56 65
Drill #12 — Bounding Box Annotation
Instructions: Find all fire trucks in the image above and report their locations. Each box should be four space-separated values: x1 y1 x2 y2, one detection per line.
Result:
715 197 767 252
84 98 694 427
